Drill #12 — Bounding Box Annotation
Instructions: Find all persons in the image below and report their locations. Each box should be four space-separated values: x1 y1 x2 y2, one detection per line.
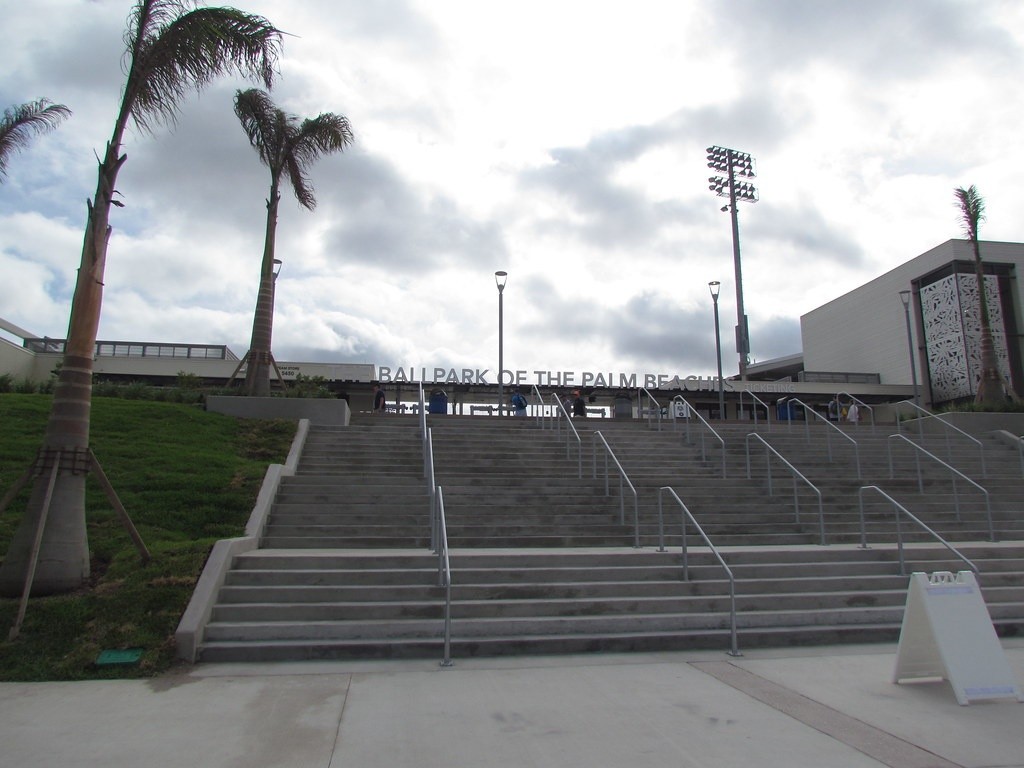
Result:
373 384 386 413
512 388 527 416
561 391 586 417
828 394 859 421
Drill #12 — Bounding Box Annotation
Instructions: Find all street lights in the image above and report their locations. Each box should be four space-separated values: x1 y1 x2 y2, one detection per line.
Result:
271 258 283 336
899 290 920 418
708 282 725 419
706 145 759 382
495 271 507 416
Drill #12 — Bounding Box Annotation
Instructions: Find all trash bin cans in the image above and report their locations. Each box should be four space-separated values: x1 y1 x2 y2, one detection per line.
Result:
429 388 448 415
777 396 795 420
